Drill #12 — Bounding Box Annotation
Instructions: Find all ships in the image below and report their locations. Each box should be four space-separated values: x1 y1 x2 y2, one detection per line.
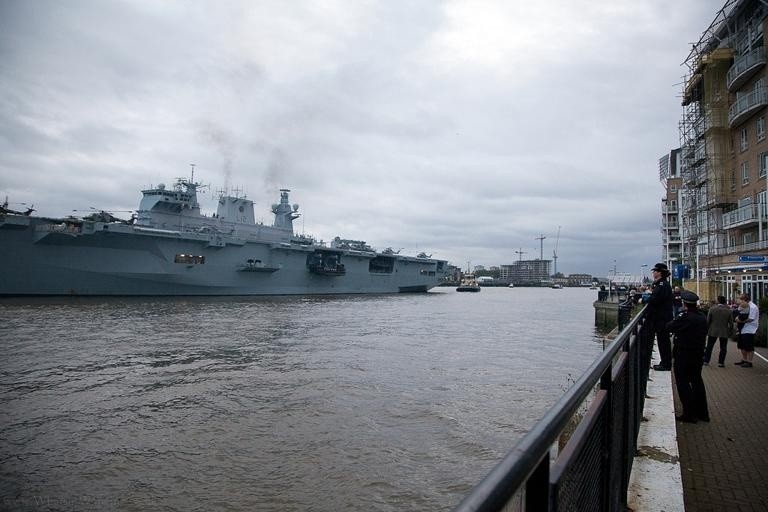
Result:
0 164 448 298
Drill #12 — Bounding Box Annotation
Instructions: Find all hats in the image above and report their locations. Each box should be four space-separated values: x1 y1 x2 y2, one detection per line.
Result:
651 263 670 272
681 290 700 305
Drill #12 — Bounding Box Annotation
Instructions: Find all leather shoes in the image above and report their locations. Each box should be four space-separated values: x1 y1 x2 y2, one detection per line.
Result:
676 415 710 424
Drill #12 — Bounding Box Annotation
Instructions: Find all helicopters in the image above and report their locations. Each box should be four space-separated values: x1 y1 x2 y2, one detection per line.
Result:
0 197 36 216
82 204 136 225
382 245 404 254
416 250 433 259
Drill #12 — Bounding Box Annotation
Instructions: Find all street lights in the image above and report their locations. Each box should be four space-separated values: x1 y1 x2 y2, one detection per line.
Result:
640 264 647 285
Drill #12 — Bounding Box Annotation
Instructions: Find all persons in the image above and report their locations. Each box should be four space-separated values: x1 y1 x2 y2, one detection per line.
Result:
696 298 738 333
735 296 750 336
733 293 759 368
598 283 653 305
671 286 682 319
701 295 733 368
637 263 674 371
665 290 710 424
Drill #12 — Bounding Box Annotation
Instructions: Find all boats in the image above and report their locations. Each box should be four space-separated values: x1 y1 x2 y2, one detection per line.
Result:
456 273 480 292
552 284 562 288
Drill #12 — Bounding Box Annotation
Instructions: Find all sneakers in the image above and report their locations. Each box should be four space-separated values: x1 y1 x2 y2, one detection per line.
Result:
718 364 724 367
734 360 753 367
653 362 671 371
